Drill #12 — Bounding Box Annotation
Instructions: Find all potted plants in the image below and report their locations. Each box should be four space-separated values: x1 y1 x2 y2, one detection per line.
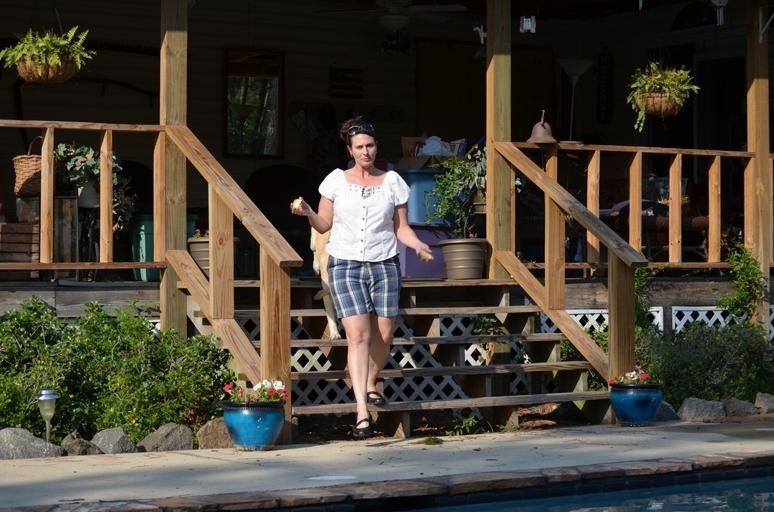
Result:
0 24 97 83
627 61 701 132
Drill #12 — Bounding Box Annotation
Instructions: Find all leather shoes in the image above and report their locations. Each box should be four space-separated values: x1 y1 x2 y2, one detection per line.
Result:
353 413 373 438
367 391 385 405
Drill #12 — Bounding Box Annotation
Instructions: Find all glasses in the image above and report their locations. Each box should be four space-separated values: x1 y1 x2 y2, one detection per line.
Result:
347 122 372 135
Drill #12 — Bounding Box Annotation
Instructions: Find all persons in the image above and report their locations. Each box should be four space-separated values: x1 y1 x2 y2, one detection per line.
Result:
291 117 434 438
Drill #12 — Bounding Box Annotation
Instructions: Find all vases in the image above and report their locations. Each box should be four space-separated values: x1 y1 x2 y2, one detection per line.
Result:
610 387 664 427
77 177 101 208
224 401 286 451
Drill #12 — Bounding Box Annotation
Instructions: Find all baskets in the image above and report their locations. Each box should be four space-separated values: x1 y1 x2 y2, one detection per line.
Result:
13 135 58 198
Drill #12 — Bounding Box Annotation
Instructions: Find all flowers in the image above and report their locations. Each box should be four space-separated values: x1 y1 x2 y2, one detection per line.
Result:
55 140 124 186
224 381 289 404
610 365 662 386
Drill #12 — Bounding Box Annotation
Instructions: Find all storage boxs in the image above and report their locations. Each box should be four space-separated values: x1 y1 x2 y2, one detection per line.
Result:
395 135 487 280
